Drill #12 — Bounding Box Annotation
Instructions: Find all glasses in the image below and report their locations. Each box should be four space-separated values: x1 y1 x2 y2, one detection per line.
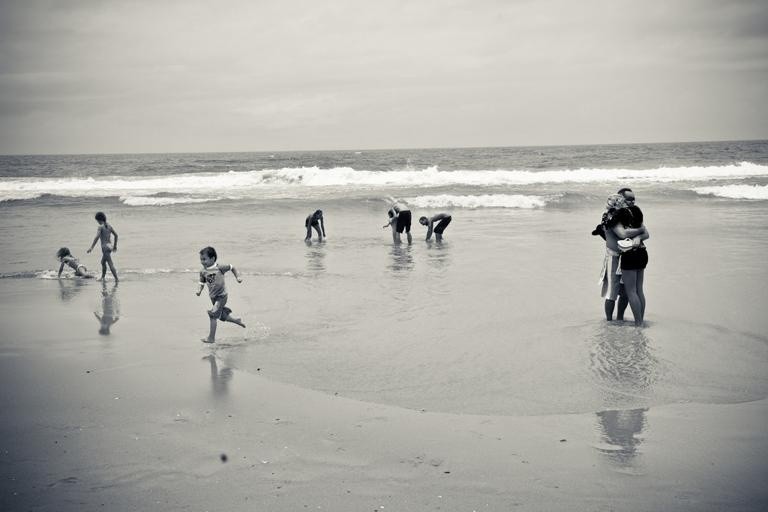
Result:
626 197 635 201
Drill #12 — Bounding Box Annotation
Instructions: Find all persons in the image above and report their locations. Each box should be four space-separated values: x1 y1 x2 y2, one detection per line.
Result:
56 247 93 279
87 211 119 283
389 203 412 243
196 245 246 343
601 187 649 321
304 209 326 240
418 212 451 239
383 210 398 240
592 193 648 327
